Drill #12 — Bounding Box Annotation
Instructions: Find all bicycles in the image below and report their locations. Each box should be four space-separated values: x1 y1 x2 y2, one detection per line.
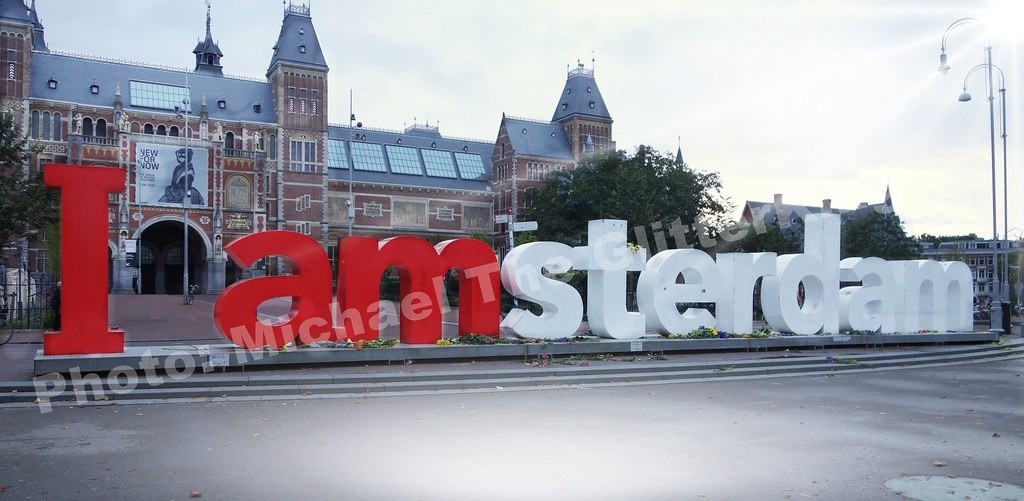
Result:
185 284 200 305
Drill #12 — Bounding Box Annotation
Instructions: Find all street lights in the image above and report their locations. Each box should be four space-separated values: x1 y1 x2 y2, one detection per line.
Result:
936 15 1005 333
344 88 367 236
175 66 190 305
957 62 1015 335
